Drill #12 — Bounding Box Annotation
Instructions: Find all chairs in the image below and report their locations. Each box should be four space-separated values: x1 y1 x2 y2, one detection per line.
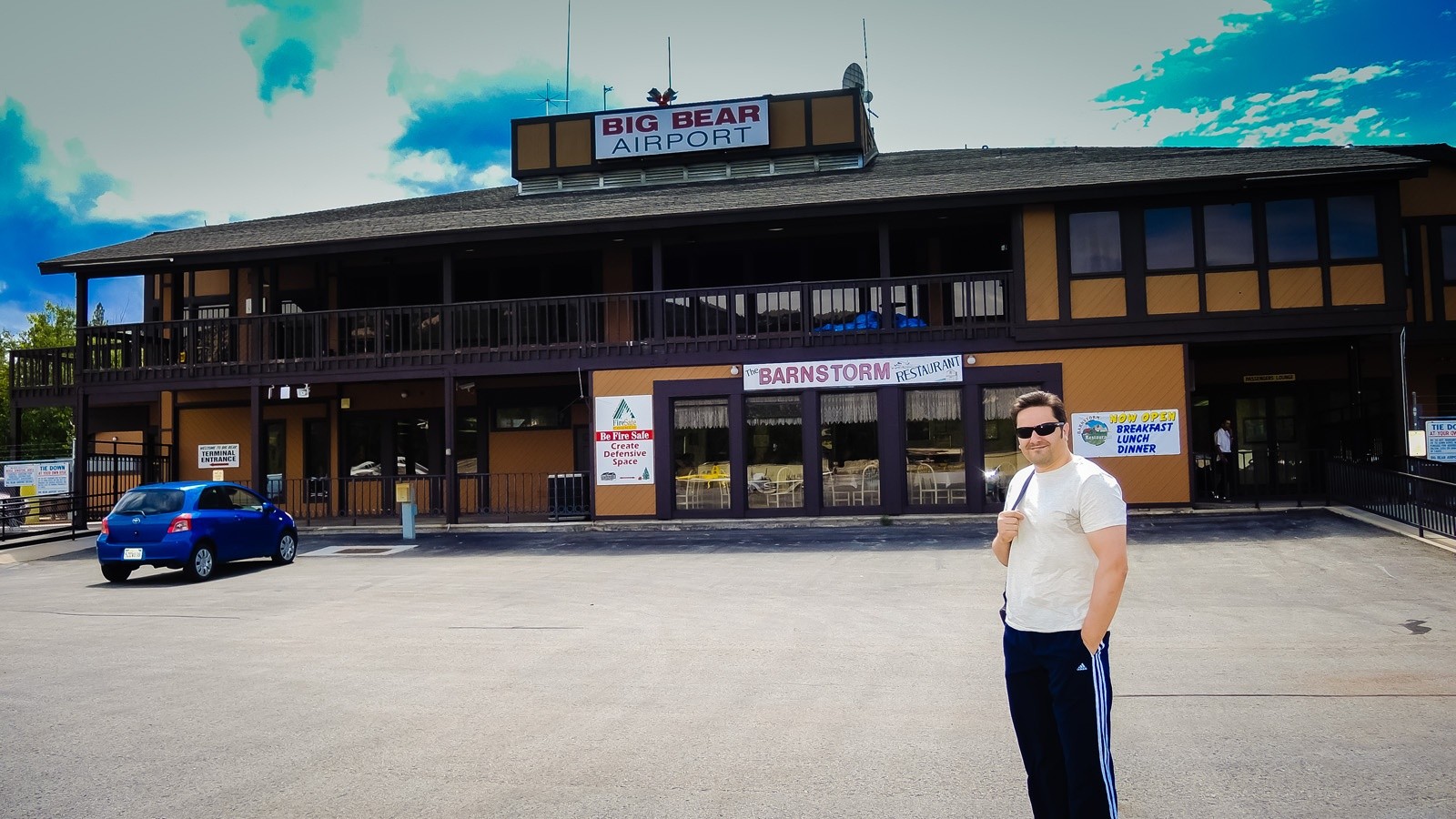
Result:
674 460 966 510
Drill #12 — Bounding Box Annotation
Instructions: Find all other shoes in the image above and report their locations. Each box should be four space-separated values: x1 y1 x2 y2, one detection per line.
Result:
1223 497 1232 503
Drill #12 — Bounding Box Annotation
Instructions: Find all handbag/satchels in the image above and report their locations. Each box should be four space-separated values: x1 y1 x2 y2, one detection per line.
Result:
1211 450 1228 470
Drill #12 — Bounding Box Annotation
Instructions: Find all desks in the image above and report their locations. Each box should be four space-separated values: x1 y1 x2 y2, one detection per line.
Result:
913 472 966 504
833 473 878 491
765 479 803 507
677 478 731 505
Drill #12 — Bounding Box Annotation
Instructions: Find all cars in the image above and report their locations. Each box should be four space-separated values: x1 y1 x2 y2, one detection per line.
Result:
93 480 299 583
0 476 22 527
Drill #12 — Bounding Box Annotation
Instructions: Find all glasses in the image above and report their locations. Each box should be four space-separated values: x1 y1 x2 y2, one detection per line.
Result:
1016 422 1065 439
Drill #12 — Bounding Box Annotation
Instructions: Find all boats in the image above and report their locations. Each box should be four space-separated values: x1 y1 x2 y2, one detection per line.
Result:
907 444 965 465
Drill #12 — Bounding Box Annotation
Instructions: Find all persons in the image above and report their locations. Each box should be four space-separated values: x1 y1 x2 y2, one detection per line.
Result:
991 391 1129 819
1210 419 1233 503
